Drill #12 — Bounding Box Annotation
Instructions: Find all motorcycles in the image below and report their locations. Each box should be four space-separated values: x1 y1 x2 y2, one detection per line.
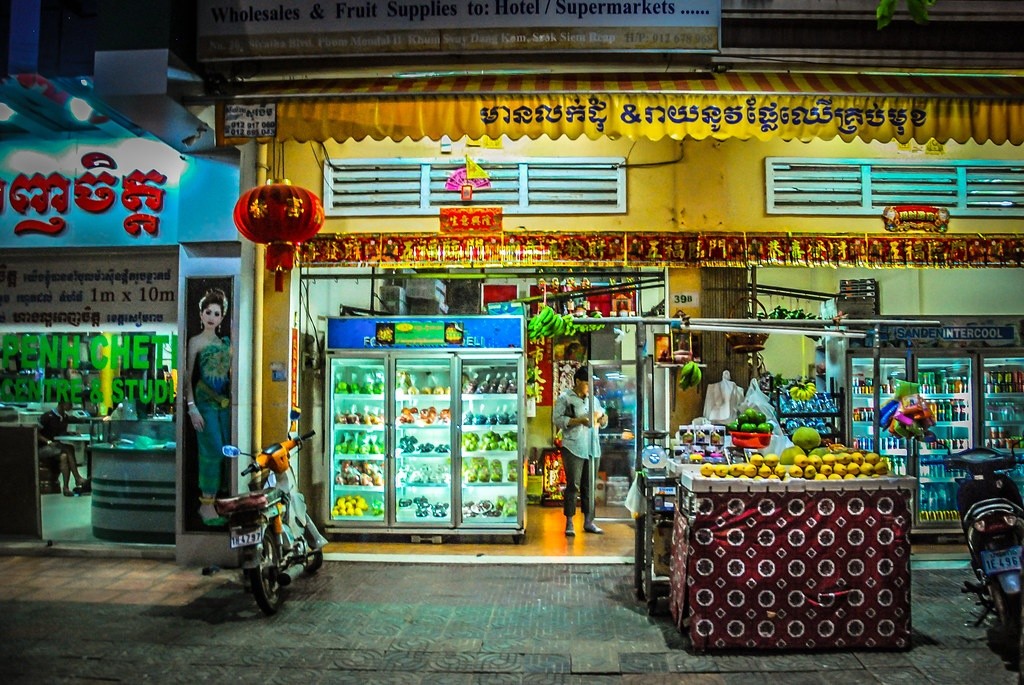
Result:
212 404 329 617
916 405 1024 651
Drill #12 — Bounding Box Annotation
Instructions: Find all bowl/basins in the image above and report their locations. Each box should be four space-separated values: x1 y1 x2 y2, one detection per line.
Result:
728 430 773 449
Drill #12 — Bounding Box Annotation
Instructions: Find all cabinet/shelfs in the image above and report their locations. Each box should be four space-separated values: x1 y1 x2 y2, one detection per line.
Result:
769 376 846 446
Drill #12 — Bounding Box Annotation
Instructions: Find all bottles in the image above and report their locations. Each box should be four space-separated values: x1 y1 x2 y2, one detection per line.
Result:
916 367 968 394
919 484 952 511
878 456 907 477
921 399 970 422
917 455 971 479
984 401 1022 422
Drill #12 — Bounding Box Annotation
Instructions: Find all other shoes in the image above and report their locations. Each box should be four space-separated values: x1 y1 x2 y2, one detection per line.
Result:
565 530 576 536
584 524 604 534
198 509 228 526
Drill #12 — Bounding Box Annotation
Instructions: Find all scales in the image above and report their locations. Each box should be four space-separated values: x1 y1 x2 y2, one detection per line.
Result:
640 429 668 476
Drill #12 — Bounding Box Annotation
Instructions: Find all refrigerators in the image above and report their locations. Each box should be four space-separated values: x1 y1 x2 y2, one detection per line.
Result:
822 314 1024 535
321 312 528 538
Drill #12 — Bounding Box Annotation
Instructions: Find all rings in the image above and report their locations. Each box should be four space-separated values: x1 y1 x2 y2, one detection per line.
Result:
195 424 197 426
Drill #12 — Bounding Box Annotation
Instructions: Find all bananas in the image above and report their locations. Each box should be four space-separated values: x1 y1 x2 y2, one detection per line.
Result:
789 383 816 400
528 307 605 339
680 361 701 390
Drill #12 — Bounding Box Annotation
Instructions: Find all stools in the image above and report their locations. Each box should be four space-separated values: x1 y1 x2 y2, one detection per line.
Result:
39 462 61 493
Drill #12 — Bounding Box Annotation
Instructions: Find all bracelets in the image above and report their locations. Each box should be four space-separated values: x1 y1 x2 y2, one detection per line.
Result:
188 402 194 405
47 440 52 444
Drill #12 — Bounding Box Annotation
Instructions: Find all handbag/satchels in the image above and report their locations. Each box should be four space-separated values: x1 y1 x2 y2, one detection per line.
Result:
564 404 576 418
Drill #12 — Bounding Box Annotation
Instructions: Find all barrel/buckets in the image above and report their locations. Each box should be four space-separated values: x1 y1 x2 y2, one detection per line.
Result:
525 473 544 505
605 476 630 507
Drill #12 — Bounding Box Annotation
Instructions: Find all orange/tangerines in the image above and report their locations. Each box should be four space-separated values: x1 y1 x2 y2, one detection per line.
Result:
727 409 774 434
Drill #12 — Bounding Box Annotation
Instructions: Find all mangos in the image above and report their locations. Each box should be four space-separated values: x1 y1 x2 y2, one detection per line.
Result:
700 452 887 480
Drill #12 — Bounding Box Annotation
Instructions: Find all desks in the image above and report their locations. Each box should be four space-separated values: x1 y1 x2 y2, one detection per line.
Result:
54 434 98 495
646 476 676 613
666 458 682 478
670 470 917 653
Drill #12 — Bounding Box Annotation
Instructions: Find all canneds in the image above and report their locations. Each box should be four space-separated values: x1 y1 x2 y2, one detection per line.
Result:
919 510 959 522
918 372 936 394
985 427 1024 450
984 371 1024 393
919 438 967 449
852 376 899 450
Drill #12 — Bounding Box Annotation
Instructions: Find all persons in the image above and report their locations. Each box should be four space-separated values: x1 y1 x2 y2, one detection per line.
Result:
552 366 609 536
185 288 233 525
38 398 86 497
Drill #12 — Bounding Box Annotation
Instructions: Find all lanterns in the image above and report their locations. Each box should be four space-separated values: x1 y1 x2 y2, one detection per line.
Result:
234 180 325 293
